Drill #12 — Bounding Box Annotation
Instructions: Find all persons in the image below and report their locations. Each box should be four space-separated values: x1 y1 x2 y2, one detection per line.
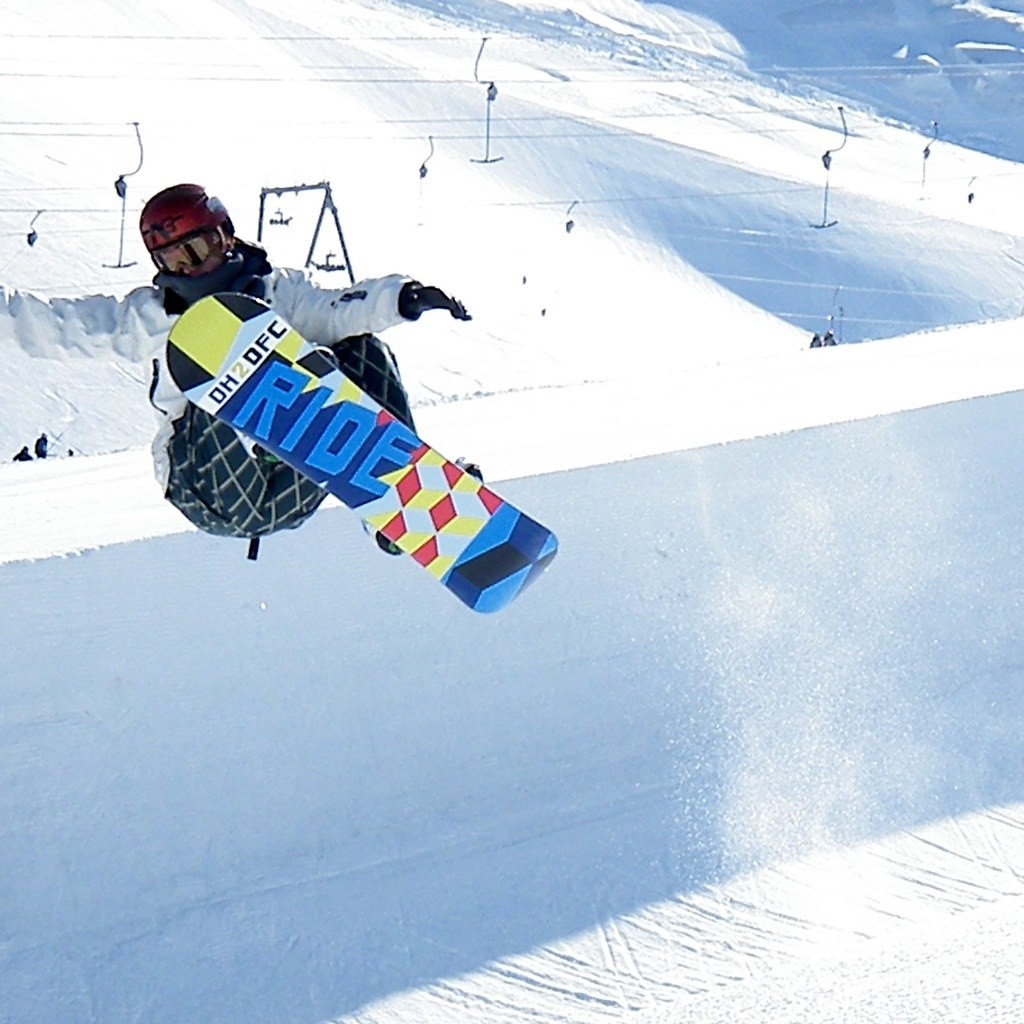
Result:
0 184 485 555
13 433 48 462
810 331 837 347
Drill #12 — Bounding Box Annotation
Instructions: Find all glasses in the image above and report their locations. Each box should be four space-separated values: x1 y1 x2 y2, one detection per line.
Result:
156 235 209 272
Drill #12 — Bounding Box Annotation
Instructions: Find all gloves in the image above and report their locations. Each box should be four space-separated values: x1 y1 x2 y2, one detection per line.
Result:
160 286 193 316
402 285 472 321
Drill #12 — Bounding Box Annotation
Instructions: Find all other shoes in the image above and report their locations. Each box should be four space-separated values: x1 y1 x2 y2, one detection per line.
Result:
361 457 484 555
234 346 340 460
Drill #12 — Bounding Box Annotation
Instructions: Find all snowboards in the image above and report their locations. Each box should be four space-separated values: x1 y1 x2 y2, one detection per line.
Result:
165 289 559 617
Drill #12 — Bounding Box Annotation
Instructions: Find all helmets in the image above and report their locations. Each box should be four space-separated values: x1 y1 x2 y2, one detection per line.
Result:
139 184 235 270
